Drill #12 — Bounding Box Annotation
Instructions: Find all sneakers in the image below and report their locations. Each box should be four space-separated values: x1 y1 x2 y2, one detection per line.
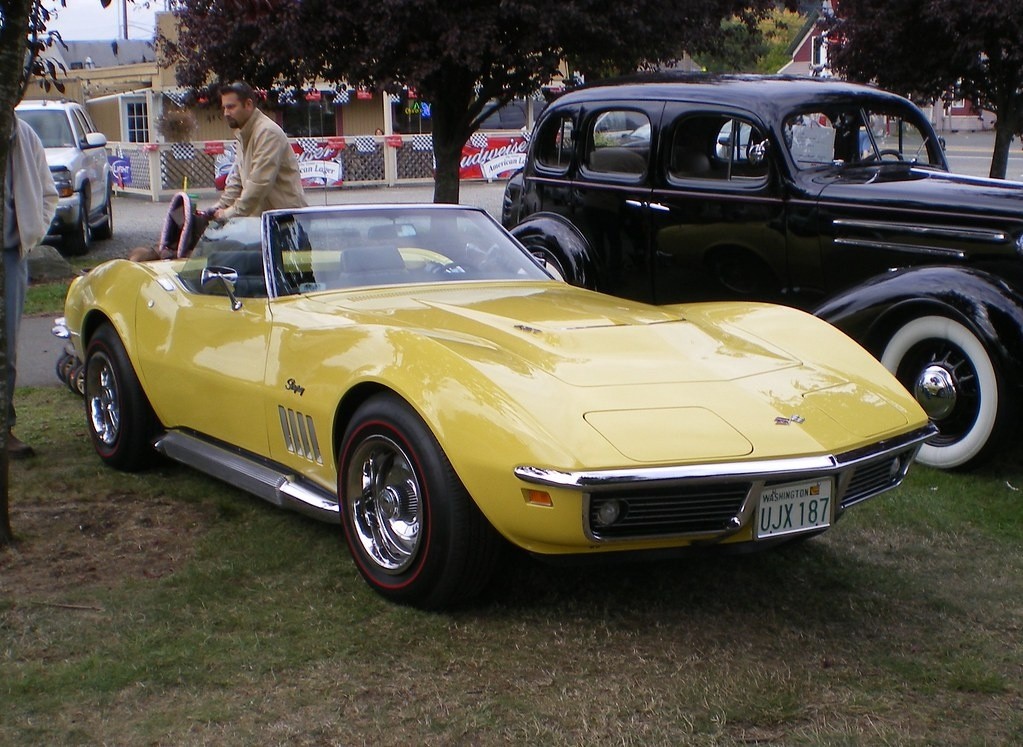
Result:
10 433 34 459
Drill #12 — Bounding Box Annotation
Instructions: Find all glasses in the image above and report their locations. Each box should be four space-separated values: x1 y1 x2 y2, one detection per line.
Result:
227 82 253 100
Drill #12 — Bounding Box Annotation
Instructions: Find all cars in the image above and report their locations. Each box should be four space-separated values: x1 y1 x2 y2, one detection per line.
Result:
621 115 835 170
480 73 1023 468
558 112 649 139
469 100 548 130
63 203 959 604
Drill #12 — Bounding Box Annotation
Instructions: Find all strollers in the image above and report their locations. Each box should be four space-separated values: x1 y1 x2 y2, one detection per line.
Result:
51 192 217 397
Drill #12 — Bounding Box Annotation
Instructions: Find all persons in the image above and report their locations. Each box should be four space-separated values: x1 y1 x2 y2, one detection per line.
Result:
4 107 60 458
129 246 160 261
206 82 308 231
374 128 384 135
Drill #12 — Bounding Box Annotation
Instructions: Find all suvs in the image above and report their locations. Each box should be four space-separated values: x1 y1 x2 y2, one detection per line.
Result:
13 98 113 251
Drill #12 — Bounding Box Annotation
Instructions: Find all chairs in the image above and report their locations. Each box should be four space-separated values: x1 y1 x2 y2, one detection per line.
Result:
589 149 646 173
676 148 718 178
206 250 289 298
339 243 410 279
